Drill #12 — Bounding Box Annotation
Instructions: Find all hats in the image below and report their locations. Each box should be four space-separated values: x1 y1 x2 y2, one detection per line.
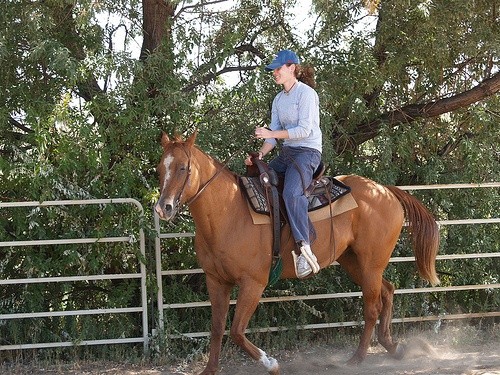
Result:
265 50 301 72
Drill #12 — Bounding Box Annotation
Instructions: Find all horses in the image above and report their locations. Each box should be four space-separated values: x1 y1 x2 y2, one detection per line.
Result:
153 127 442 374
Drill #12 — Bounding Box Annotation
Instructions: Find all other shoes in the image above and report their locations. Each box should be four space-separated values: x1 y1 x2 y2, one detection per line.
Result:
297 253 317 278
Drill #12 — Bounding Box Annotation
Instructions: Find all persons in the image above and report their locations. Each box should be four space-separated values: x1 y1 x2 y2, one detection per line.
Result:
243 50 323 279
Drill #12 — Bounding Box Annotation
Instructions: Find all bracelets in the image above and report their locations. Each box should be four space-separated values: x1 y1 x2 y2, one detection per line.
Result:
257 150 263 157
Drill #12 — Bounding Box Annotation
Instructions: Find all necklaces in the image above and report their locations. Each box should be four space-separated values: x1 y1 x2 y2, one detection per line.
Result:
284 81 297 94
